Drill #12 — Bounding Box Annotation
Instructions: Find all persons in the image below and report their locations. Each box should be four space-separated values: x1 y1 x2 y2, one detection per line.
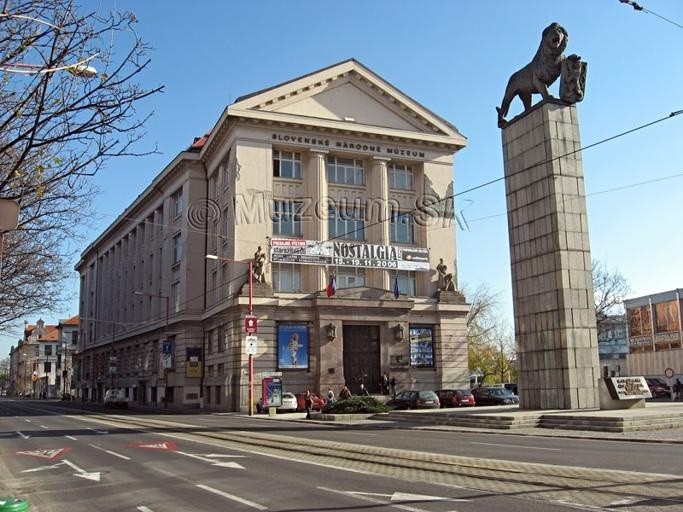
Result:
254 245 265 269
323 389 336 404
304 389 313 419
436 257 447 289
338 386 351 399
356 383 369 397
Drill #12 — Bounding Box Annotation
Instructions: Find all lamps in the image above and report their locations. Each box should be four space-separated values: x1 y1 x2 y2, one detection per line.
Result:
394 324 404 341
326 323 336 341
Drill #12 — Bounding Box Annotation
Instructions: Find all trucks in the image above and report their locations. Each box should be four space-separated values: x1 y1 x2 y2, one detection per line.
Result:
104 388 128 409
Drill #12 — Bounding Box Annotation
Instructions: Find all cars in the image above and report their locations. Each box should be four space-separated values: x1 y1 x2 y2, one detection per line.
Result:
646 378 670 399
386 383 519 409
256 391 324 413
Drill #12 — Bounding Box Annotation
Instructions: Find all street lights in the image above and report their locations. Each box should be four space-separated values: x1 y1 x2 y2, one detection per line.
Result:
205 253 253 416
133 291 169 409
0 63 96 77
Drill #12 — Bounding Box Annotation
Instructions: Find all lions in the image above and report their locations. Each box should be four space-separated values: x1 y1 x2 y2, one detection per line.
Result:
498 23 581 128
